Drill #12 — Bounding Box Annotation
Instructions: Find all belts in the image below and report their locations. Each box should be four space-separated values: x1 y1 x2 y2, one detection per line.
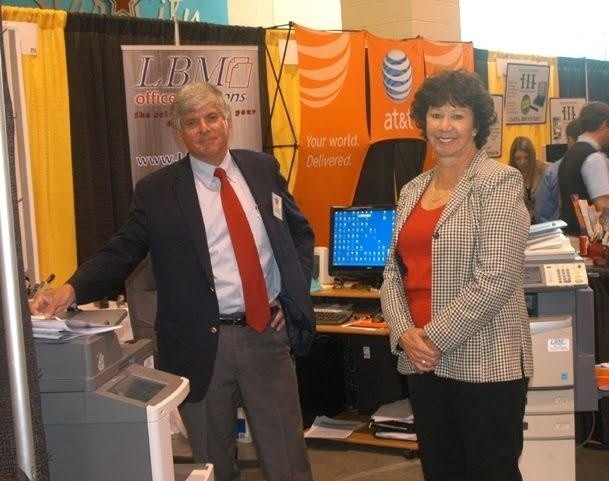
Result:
218 306 278 326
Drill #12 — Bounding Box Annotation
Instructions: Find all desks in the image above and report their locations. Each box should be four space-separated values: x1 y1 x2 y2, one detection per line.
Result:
310 285 389 410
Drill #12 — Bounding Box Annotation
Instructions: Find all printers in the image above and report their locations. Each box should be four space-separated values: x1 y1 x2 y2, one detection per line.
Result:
522 251 599 415
29 309 216 481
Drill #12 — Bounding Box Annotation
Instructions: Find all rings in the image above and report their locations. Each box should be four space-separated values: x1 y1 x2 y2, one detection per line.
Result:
422 360 427 367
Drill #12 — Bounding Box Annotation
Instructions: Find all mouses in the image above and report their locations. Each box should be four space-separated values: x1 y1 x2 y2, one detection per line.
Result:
372 313 384 323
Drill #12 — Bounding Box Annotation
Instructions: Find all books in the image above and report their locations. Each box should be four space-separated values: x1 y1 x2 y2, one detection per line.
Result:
30 315 124 341
524 219 577 256
371 399 419 443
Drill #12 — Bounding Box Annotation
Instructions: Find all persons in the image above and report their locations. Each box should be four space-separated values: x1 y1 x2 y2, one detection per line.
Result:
532 118 581 222
379 68 535 479
508 135 554 224
557 101 609 450
27 83 316 481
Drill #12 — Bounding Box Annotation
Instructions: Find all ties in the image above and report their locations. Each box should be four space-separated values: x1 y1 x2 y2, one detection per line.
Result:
215 168 270 335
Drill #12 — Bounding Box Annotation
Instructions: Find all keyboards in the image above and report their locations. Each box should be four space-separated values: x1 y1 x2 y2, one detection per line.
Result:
313 311 352 325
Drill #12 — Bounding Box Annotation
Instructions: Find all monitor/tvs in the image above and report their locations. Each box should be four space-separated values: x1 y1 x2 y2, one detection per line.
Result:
329 205 397 289
